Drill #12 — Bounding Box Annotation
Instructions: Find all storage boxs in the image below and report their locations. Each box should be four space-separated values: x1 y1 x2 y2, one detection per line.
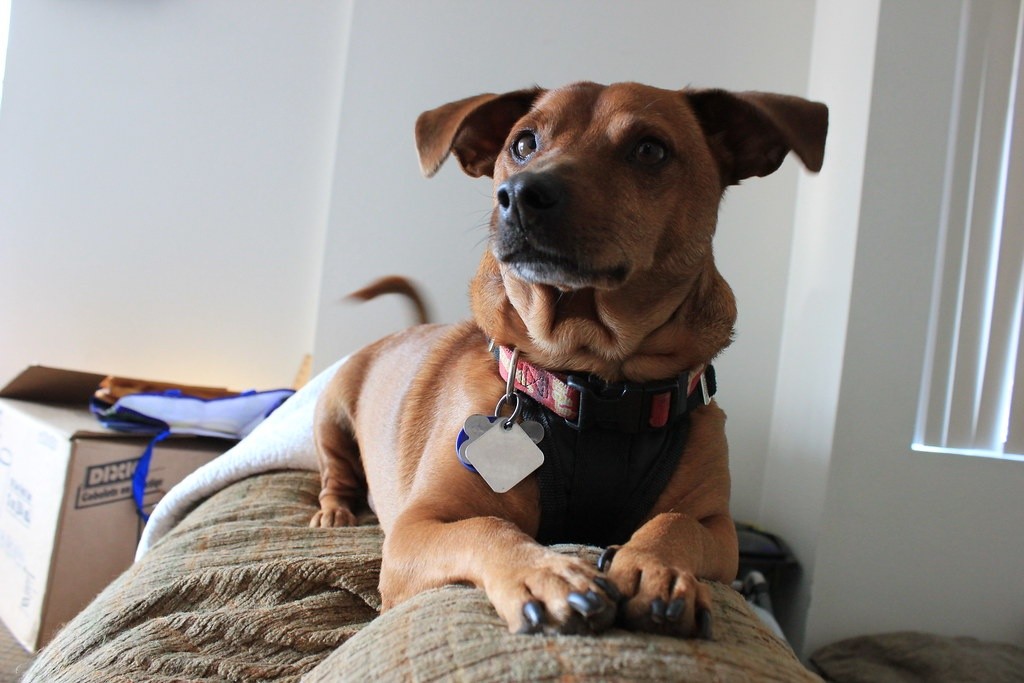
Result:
0 364 245 653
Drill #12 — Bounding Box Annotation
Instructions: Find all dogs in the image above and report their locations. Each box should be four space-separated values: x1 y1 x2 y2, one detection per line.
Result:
311 79 830 640
